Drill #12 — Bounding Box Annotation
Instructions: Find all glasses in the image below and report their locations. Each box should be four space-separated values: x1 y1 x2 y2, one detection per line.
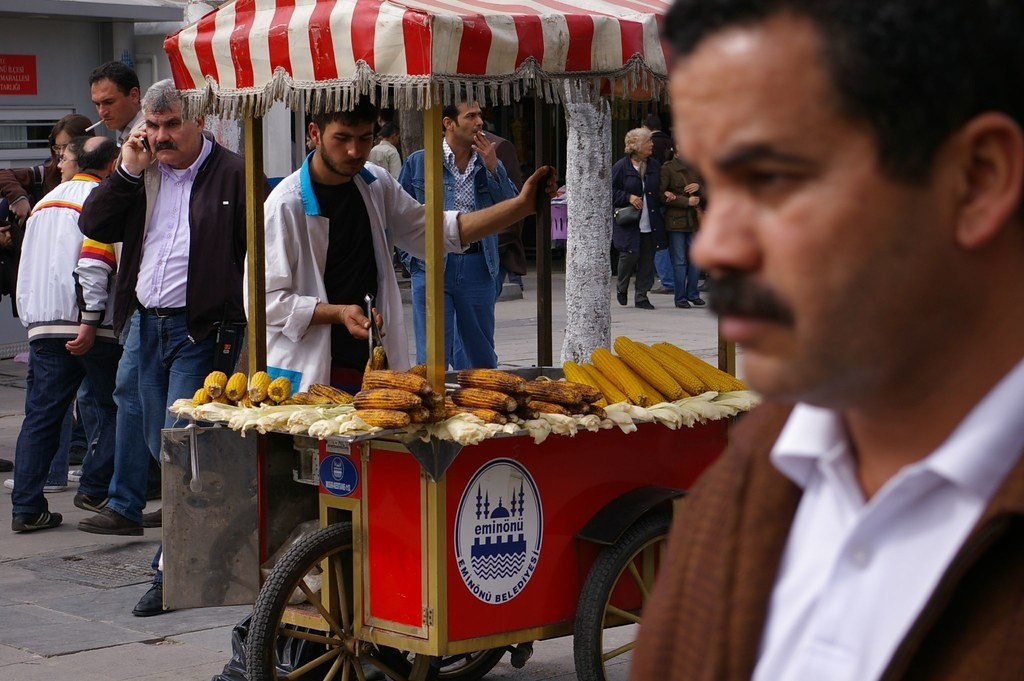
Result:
51 145 68 152
59 156 79 163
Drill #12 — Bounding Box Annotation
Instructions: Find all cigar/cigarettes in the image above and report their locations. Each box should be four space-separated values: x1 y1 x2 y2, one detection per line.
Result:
480 131 486 136
85 118 105 132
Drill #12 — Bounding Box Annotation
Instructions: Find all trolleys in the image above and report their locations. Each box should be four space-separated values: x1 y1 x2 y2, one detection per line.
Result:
164 0 736 680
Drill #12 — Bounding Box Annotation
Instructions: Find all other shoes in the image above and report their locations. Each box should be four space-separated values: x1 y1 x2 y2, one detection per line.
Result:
651 284 674 294
687 296 705 306
4 479 65 492
617 285 628 305
698 278 710 291
68 470 83 481
0 459 13 472
635 299 655 310
674 301 691 308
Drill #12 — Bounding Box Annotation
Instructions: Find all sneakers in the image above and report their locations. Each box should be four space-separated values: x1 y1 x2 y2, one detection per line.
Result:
143 509 162 527
73 491 110 513
12 510 63 531
77 507 144 536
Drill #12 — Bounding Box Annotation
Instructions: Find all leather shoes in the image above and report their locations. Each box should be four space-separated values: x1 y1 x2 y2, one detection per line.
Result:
131 581 165 615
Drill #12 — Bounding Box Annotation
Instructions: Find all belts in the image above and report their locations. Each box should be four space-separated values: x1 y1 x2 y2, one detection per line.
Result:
145 305 187 317
464 241 482 253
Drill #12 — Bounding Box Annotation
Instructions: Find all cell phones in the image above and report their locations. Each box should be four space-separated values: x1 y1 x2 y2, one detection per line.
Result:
140 136 151 151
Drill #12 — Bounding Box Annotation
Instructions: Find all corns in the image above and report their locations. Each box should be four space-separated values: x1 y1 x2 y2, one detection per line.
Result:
564 336 748 407
192 371 292 408
290 346 444 429
444 367 608 427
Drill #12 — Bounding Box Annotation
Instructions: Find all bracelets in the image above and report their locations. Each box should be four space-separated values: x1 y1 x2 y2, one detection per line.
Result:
341 305 348 323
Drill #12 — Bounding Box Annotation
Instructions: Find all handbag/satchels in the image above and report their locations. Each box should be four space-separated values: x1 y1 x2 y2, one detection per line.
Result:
614 205 640 223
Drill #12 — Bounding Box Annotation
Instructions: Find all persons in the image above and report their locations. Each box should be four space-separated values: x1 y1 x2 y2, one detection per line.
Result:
364 106 411 280
0 60 165 536
241 82 558 392
77 72 273 467
393 85 522 371
306 133 316 150
626 1 1024 681
610 124 677 310
650 127 708 309
